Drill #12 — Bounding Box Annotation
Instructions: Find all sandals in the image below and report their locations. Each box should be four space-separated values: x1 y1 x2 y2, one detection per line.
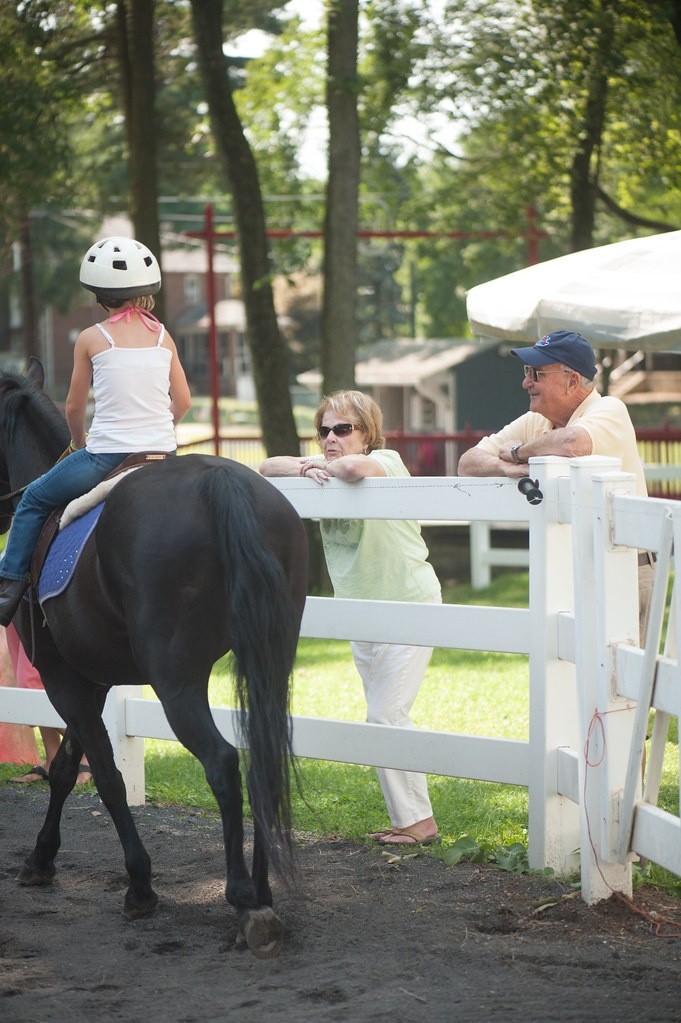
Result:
6 765 50 784
76 765 93 785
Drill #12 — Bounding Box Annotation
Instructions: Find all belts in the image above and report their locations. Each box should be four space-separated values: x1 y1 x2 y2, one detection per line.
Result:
638 552 656 564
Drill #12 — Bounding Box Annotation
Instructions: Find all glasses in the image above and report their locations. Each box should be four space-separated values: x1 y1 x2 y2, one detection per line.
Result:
319 423 365 439
524 366 572 382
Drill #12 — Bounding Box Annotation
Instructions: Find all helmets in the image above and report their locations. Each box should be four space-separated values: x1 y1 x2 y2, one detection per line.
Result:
79 237 162 300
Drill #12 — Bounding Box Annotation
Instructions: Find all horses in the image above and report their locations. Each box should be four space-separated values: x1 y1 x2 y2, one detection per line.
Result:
0 354 311 958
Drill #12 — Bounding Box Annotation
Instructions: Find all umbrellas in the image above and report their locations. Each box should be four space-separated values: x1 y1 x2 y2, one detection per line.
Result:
465 229 681 353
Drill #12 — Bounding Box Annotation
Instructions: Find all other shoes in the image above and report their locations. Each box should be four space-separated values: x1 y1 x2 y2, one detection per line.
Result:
0 577 28 627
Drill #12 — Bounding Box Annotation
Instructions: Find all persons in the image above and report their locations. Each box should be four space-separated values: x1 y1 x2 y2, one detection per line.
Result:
453 328 654 644
3 620 94 787
261 387 444 847
0 235 192 628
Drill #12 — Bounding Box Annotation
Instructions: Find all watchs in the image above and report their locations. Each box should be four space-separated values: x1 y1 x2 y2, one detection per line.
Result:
511 440 529 465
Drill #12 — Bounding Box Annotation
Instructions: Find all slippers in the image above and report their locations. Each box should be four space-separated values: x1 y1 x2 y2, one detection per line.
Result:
368 830 391 838
381 828 441 846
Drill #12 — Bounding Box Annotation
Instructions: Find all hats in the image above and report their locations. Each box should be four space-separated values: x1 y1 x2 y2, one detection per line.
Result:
511 330 597 380
